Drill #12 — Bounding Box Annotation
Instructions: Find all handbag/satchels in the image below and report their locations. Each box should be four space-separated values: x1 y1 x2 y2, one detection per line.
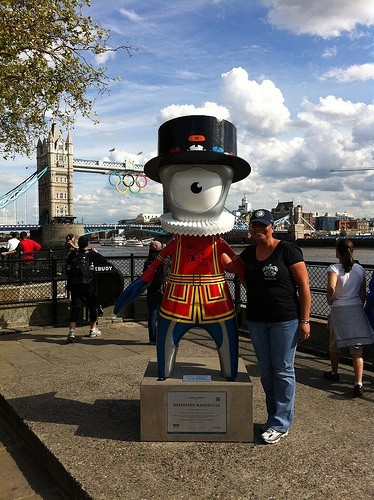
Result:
161 255 172 295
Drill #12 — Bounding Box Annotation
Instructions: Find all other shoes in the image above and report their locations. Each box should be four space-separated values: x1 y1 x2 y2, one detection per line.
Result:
149 342 156 345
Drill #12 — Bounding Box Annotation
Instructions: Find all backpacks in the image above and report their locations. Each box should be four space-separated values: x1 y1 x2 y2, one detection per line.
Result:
71 250 94 284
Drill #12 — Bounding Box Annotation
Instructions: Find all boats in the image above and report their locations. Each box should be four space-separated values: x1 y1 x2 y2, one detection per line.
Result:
126 239 143 248
100 237 126 246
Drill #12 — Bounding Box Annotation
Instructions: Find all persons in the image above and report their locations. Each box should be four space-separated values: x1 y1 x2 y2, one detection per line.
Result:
66 235 107 343
323 239 374 396
1 232 79 284
143 240 171 346
214 209 311 444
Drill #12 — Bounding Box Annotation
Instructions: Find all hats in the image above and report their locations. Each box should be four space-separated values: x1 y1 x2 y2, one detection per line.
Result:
149 241 162 251
250 209 274 226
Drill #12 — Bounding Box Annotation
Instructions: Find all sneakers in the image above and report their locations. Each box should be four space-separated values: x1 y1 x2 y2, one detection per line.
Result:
353 382 364 396
261 427 289 443
90 328 103 339
261 424 274 431
67 331 75 343
323 370 340 382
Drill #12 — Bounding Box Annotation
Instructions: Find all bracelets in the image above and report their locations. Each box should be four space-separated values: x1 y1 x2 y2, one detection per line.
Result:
299 320 310 323
